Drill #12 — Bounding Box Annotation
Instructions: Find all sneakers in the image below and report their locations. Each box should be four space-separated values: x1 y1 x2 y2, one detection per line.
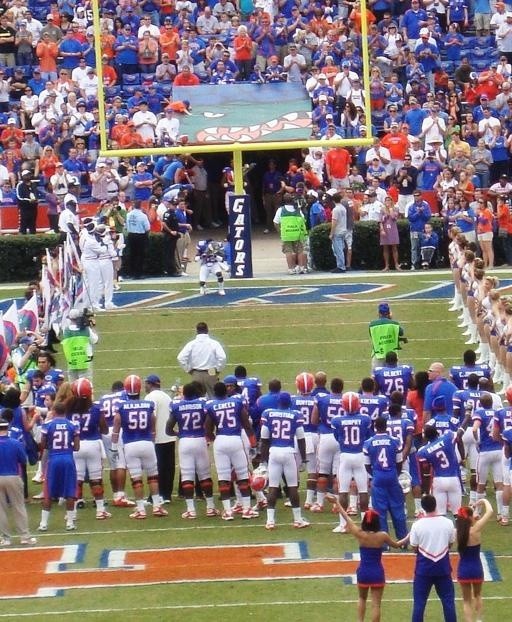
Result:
21 537 37 545
1 538 11 546
33 463 509 532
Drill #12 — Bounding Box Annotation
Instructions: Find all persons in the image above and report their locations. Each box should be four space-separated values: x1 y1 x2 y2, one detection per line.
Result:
1 1 511 621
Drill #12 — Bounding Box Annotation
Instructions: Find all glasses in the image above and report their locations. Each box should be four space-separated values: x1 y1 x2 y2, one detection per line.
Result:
306 66 512 169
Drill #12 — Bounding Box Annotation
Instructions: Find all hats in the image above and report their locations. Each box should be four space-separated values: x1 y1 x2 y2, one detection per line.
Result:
55 161 63 168
66 200 77 206
420 31 430 38
7 5 176 155
146 375 160 387
21 169 31 177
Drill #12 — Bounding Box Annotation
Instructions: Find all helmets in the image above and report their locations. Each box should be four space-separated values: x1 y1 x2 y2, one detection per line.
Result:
70 378 93 401
295 373 317 397
123 374 143 398
342 391 363 415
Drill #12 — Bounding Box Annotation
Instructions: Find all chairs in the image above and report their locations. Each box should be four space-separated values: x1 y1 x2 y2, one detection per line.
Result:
103 71 171 110
78 185 97 203
1 65 41 79
440 34 499 72
198 21 252 46
22 0 56 21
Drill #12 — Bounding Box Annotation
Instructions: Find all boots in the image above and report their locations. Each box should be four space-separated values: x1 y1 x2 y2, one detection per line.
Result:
449 285 512 396
